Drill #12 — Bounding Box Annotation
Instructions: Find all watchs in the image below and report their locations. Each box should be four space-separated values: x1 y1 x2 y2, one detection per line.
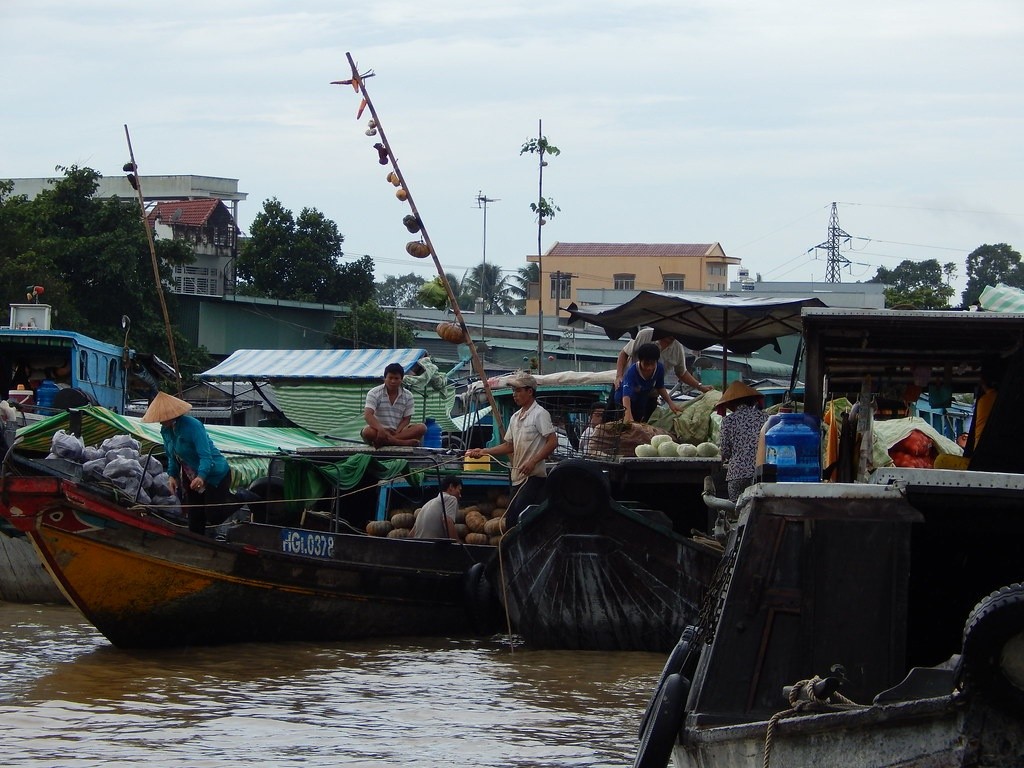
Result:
698 382 703 390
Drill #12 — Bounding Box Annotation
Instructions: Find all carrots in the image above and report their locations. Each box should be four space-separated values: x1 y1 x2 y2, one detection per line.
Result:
356 99 365 120
352 79 358 93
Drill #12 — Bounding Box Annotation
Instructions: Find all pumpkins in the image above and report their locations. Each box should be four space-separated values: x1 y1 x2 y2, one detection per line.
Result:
387 172 400 186
634 435 720 457
402 215 420 233
396 189 407 201
365 489 510 547
436 320 466 344
405 240 431 258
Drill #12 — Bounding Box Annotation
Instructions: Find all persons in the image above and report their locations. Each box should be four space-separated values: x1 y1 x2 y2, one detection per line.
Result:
713 381 769 506
465 371 558 530
614 343 686 424
141 391 232 538
578 401 607 454
612 322 714 394
405 475 465 545
360 361 428 449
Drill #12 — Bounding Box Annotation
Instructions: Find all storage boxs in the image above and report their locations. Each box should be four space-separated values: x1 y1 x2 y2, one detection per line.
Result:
9 390 34 404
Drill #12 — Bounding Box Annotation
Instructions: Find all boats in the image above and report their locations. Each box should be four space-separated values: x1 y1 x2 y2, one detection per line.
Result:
0 321 805 658
633 302 1024 768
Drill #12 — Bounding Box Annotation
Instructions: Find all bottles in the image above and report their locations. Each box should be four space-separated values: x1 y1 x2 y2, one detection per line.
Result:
422 418 442 448
763 406 821 460
35 380 61 416
763 412 823 482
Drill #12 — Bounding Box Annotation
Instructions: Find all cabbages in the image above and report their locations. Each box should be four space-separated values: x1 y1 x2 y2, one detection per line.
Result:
417 278 450 311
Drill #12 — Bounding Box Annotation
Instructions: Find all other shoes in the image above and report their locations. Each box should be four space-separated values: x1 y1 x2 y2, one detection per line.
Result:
235 487 261 502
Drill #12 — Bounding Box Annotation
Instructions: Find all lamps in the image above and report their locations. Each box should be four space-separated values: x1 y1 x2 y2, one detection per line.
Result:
928 376 952 409
857 356 892 374
120 314 131 369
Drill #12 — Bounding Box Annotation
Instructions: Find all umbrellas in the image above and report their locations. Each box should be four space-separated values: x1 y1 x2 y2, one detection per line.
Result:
559 292 829 396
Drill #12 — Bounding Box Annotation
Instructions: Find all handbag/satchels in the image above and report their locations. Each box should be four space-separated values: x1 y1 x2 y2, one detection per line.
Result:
45 429 181 519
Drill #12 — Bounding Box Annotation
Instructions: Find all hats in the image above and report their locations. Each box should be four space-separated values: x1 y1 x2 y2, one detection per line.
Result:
505 373 537 391
141 391 192 423
714 380 764 409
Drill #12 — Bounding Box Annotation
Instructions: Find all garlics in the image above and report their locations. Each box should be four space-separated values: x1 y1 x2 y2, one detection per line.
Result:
365 120 376 136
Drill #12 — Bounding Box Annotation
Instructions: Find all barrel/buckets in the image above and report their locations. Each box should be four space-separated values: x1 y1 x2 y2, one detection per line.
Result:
8 390 36 415
